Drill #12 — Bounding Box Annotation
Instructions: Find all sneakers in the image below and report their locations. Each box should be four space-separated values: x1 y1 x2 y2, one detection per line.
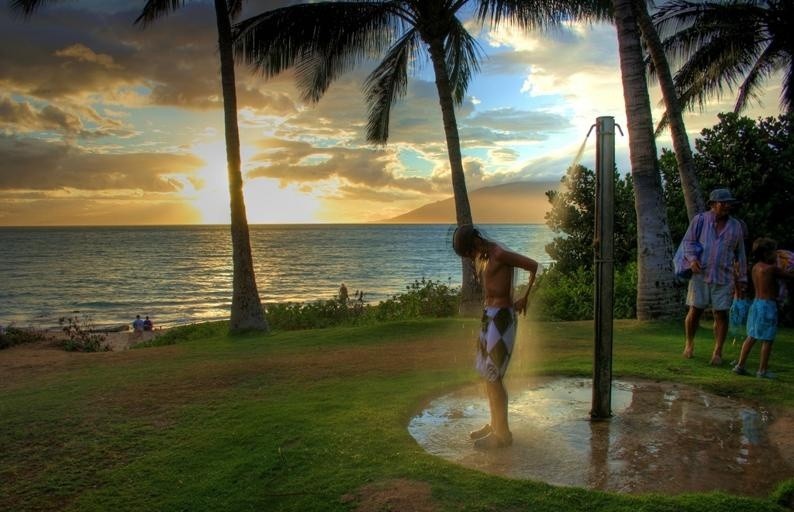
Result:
733 365 744 373
471 425 493 439
475 431 514 449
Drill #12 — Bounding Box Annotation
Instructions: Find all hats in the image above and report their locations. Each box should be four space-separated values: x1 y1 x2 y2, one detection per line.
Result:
710 189 737 202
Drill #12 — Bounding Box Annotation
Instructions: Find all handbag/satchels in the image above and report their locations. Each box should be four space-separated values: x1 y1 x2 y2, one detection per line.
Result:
673 238 703 280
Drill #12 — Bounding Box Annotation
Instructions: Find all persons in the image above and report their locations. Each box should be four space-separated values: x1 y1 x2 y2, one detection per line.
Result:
144 316 153 330
733 239 794 379
452 223 538 451
340 283 348 299
133 315 144 330
681 189 748 366
